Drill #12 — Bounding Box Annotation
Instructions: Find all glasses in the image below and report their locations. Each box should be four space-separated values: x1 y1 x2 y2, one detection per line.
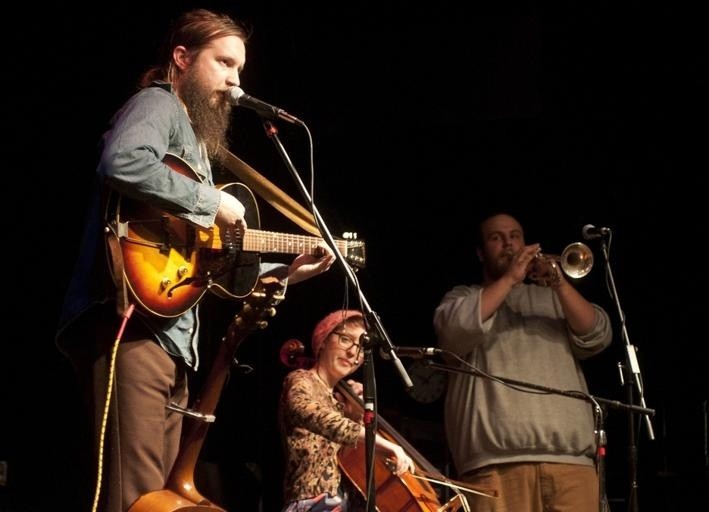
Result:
331 331 365 359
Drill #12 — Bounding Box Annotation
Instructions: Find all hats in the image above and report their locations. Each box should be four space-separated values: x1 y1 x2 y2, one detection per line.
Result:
311 309 364 361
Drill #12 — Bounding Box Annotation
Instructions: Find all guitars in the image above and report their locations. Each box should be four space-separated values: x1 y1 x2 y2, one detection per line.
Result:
96 152 367 319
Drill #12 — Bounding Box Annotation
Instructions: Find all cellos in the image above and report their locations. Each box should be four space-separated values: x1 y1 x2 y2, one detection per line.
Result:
280 337 448 511
122 277 287 512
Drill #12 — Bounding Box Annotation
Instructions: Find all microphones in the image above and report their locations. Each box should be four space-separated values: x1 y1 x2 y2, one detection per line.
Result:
392 345 443 358
225 86 304 127
582 224 611 240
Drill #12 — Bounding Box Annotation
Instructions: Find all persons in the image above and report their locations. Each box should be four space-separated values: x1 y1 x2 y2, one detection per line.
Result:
434 203 615 511
82 10 337 511
275 307 417 511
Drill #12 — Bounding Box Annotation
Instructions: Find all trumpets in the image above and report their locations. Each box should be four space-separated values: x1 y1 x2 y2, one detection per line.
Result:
507 241 594 287
